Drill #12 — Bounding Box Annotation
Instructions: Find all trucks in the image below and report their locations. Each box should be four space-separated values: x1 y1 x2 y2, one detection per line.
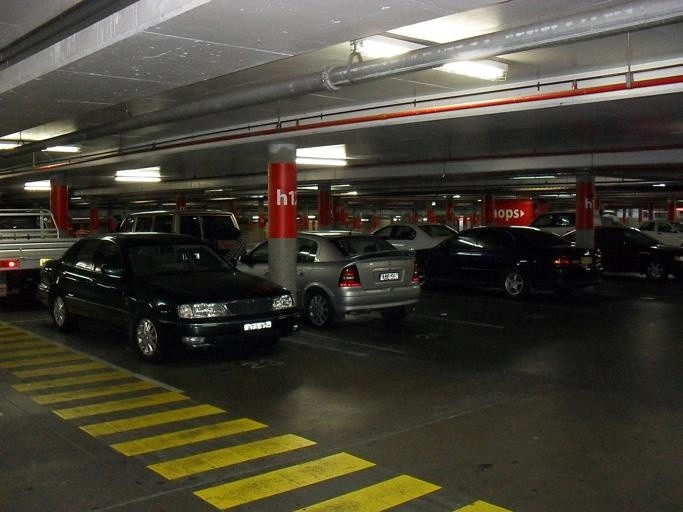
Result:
0 206 84 299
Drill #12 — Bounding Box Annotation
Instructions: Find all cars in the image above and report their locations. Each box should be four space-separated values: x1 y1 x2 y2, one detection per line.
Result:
37 230 301 358
228 228 423 328
369 221 458 253
635 219 682 246
561 226 682 280
411 225 604 301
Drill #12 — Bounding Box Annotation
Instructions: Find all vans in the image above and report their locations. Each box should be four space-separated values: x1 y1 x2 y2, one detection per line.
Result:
528 209 618 236
118 207 241 265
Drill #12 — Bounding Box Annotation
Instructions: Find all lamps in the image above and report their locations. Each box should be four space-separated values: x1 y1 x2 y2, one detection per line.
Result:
435 60 509 81
